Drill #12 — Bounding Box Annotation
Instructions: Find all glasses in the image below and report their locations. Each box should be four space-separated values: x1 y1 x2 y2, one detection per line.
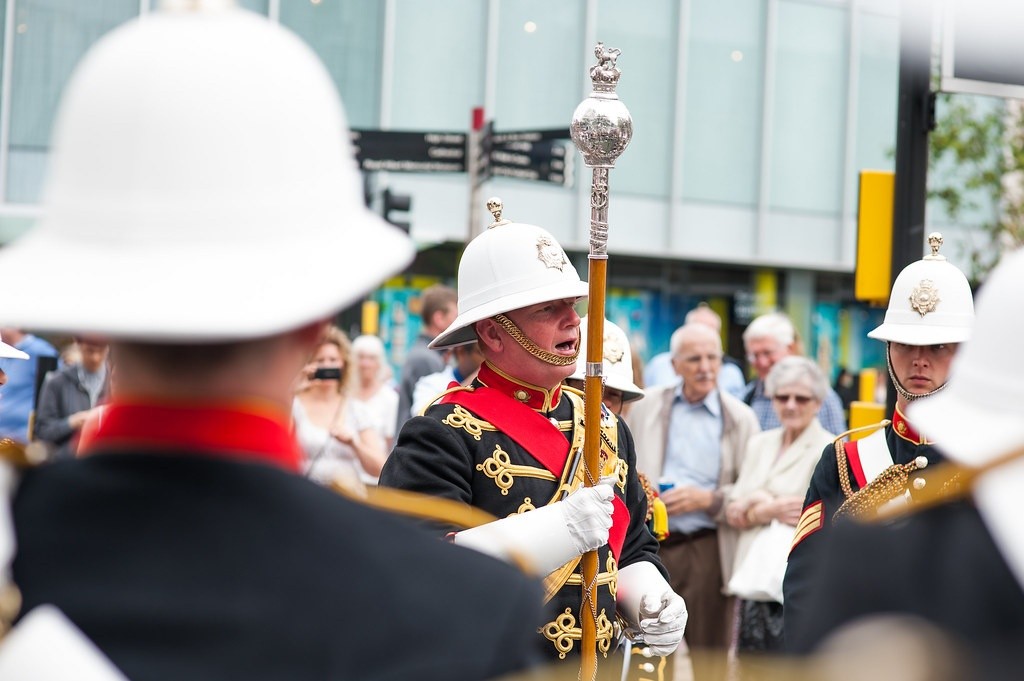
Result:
773 394 816 405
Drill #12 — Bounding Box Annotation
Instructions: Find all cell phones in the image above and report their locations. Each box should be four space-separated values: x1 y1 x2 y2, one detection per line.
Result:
315 368 341 379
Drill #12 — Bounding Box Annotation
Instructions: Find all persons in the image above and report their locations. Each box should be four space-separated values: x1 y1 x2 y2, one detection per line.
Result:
377 198 688 681
782 248 1024 681
0 286 849 681
783 232 975 598
0 0 545 681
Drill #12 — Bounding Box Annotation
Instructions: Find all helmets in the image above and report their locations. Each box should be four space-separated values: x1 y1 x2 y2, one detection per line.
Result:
428 197 591 350
866 233 975 346
904 250 1024 472
566 311 644 403
0 0 418 339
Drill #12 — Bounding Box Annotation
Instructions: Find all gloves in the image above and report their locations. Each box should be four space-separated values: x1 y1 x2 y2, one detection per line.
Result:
562 477 617 554
640 582 688 656
